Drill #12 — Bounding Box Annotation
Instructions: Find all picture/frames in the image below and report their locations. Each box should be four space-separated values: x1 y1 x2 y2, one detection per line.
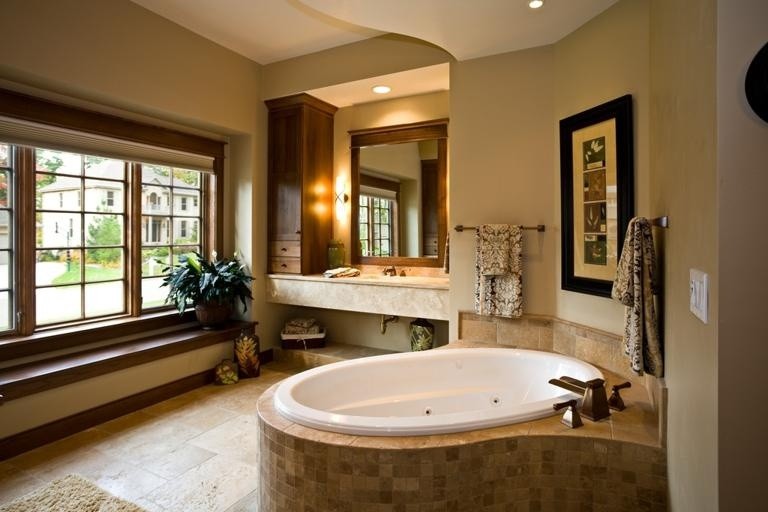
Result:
559 93 634 299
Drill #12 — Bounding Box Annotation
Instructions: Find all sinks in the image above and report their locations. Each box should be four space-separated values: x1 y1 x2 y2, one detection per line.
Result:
347 275 392 280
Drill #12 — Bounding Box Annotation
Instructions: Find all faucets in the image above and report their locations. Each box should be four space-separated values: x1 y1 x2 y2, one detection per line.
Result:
549 376 612 421
383 265 397 276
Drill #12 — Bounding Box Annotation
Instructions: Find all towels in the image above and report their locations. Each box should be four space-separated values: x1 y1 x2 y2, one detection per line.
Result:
474 224 527 320
323 267 360 277
611 216 664 379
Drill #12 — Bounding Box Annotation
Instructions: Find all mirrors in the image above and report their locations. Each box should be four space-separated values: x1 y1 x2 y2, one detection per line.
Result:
347 117 449 268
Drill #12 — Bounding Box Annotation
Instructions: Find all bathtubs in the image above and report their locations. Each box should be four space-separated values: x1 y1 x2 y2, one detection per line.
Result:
274 347 605 438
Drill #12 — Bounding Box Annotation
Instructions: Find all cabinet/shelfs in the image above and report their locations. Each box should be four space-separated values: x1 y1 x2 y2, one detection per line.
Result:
421 159 439 255
263 93 340 273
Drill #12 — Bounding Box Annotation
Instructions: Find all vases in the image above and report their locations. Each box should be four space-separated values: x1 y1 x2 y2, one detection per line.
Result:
328 240 344 268
234 320 261 380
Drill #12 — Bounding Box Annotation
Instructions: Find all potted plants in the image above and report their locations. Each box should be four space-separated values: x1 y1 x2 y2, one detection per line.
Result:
154 250 256 329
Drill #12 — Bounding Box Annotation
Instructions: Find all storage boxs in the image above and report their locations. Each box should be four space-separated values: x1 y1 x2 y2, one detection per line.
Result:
281 326 327 349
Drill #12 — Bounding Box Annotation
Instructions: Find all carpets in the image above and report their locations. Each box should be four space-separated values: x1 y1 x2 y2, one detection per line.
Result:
0 473 150 512
